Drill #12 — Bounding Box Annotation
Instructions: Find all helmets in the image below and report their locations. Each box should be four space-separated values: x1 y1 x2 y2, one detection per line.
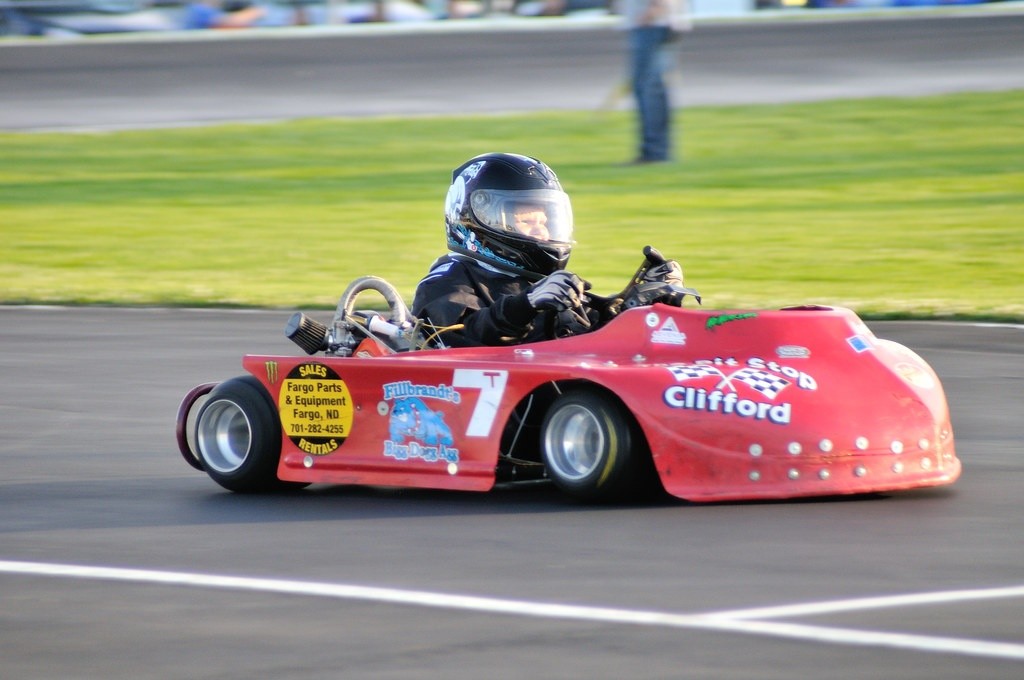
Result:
445 153 573 278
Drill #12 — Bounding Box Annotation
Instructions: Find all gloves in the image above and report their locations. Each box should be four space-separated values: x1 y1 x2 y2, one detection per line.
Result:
635 260 688 290
528 272 593 313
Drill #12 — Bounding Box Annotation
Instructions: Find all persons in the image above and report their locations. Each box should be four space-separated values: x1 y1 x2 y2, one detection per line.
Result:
628 0 692 162
0 0 627 45
412 151 686 350
